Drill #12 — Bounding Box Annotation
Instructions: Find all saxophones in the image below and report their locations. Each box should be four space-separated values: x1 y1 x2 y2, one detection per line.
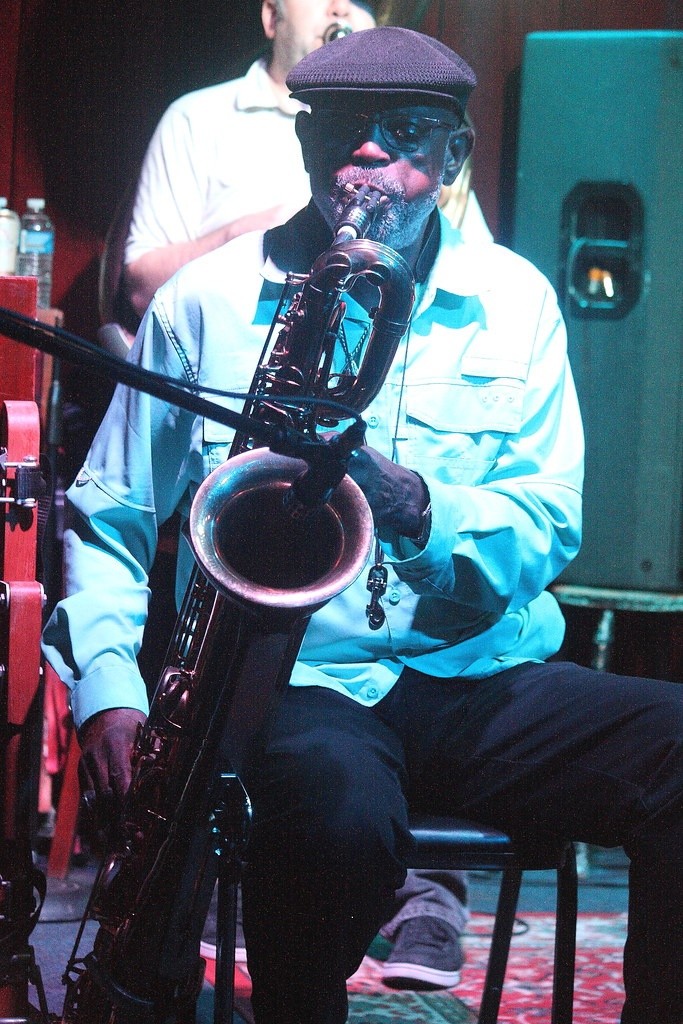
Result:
59 178 419 1011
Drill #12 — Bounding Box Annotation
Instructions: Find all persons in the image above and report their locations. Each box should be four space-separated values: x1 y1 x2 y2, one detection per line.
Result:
41 24 683 1024
125 1 499 988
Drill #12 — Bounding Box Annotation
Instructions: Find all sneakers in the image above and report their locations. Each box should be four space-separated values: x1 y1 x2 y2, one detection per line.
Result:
384 913 466 988
199 884 247 962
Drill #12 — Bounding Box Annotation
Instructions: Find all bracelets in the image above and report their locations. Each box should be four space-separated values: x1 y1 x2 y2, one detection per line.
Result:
400 502 434 548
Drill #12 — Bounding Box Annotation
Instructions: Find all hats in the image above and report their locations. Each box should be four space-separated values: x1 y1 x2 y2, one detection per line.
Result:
286 26 476 126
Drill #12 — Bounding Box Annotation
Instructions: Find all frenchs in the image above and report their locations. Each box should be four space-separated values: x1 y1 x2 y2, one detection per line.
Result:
96 0 477 362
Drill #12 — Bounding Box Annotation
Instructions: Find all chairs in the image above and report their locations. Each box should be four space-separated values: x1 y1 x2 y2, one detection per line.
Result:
139 520 580 1024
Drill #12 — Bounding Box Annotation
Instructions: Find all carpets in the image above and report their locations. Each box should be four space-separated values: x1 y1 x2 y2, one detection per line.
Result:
193 909 630 1024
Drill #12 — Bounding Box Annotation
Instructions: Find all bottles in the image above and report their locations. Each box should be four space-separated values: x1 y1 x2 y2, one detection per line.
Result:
0 195 55 313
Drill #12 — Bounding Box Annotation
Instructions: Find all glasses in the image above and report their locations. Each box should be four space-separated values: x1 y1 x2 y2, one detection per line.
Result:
311 108 458 154
